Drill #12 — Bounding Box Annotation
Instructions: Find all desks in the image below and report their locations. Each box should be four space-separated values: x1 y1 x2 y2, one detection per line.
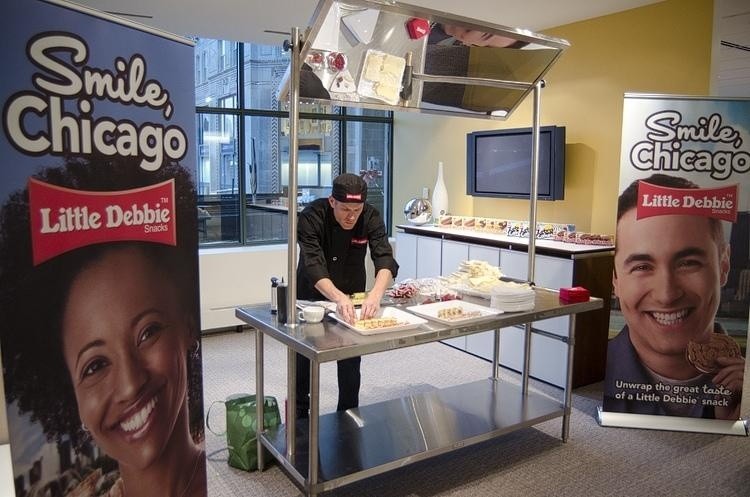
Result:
234 278 604 496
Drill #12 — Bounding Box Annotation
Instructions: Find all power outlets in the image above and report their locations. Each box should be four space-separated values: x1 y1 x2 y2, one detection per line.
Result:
423 188 429 199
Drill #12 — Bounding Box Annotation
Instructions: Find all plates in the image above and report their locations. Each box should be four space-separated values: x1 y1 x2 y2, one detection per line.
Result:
406 300 503 324
328 307 428 336
491 285 536 313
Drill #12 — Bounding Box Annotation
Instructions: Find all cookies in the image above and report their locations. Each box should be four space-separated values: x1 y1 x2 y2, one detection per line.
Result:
685 333 742 375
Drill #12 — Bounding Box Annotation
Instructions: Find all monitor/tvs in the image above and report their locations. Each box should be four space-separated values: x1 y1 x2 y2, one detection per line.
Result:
466 125 565 202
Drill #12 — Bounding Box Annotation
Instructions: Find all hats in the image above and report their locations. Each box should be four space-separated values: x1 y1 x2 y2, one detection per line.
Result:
331 173 368 203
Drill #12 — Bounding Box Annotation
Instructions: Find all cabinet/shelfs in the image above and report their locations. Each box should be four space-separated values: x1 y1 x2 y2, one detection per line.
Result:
396 222 614 391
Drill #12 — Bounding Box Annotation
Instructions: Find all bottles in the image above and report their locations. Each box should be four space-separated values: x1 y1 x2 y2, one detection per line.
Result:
432 161 449 225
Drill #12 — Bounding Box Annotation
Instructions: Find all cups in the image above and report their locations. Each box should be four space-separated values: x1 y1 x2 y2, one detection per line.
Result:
269 281 295 323
297 306 325 324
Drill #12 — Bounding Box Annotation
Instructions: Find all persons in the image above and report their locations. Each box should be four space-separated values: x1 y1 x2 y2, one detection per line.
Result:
297 174 398 417
0 156 206 497
427 23 532 49
603 174 745 420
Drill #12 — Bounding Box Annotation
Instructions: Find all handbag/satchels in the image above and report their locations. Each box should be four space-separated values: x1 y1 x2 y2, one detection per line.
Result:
226 394 280 471
